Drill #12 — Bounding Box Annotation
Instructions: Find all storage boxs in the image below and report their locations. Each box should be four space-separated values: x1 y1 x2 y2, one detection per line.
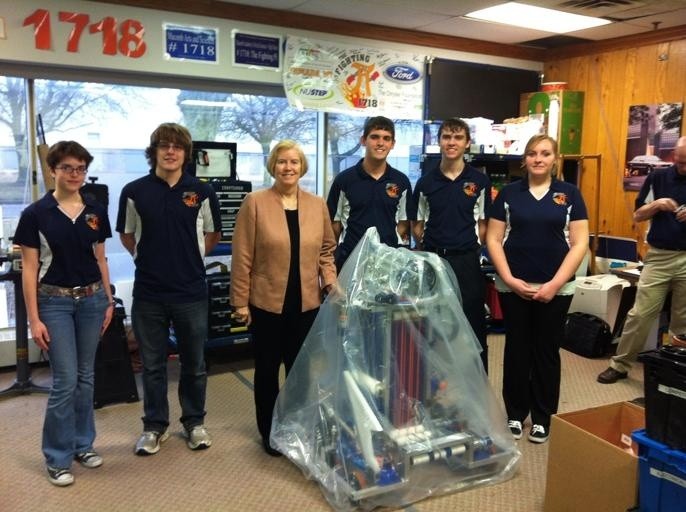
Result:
544 401 645 511
631 429 686 512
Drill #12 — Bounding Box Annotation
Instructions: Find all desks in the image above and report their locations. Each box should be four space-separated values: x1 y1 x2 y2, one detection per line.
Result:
609 268 640 286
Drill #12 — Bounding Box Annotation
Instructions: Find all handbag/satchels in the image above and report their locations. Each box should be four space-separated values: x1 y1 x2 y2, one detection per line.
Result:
559 311 613 359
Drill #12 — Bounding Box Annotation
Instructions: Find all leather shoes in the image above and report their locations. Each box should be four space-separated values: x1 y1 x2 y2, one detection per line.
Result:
596 366 628 384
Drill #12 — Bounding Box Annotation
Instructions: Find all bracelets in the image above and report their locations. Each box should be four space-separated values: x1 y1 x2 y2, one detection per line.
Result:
109 300 115 306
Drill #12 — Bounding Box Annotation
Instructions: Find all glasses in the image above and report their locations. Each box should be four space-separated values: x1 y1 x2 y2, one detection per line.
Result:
54 164 87 175
157 141 185 152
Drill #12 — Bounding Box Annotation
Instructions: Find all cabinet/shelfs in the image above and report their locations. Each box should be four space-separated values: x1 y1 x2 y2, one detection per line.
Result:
419 154 525 336
557 153 602 275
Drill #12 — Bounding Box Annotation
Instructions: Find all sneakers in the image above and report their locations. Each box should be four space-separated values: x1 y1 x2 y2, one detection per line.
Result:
261 431 283 457
181 424 212 450
75 448 103 468
46 465 74 486
136 426 169 454
528 422 551 443
507 418 524 439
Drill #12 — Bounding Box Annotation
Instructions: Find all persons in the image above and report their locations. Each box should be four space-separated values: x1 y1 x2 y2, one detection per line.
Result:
485 136 589 443
597 136 686 383
326 116 413 278
13 141 114 487
229 140 343 458
411 119 498 376
115 122 222 455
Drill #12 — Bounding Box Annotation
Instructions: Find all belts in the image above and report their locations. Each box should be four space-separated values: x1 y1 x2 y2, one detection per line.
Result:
431 246 464 257
37 280 103 300
